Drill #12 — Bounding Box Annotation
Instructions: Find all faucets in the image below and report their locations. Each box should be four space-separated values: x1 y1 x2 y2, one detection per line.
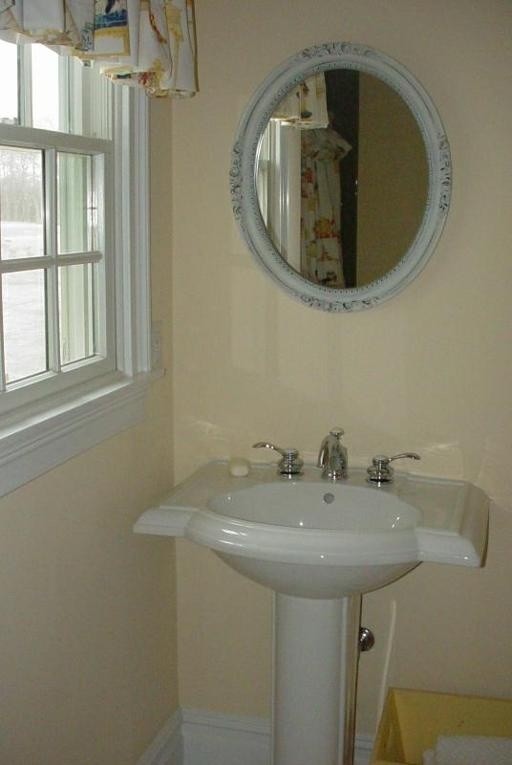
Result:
316 428 345 478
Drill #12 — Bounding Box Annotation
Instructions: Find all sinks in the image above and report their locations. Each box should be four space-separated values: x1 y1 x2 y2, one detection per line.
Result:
208 479 422 600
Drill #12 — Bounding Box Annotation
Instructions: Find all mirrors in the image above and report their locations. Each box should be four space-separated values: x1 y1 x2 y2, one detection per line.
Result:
230 41 451 315
